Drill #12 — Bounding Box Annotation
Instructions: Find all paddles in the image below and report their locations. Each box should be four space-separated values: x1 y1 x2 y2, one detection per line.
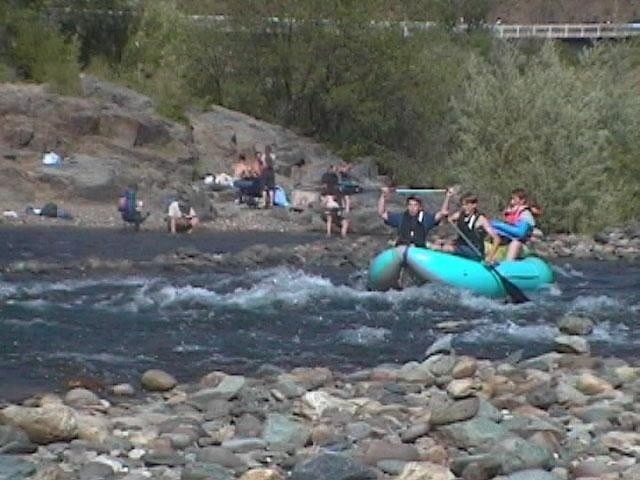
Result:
449 219 532 304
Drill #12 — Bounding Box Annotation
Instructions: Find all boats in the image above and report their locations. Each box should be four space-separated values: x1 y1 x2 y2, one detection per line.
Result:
368 245 553 301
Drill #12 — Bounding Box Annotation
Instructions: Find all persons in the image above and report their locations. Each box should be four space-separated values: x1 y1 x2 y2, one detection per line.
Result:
320 163 340 194
373 185 454 248
448 191 502 268
166 197 199 235
234 155 249 184
320 190 351 239
260 144 277 210
486 188 537 263
252 151 265 180
117 190 151 231
